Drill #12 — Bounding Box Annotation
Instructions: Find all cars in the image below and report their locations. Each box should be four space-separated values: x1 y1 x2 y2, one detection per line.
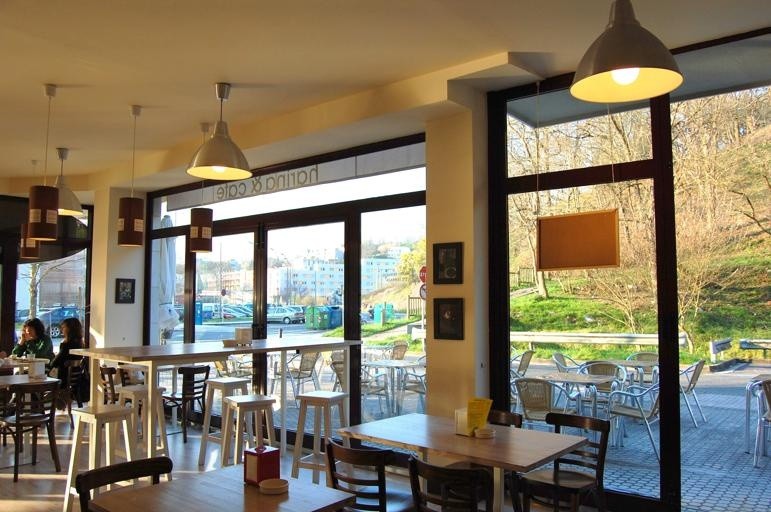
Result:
167 301 306 324
16 304 86 338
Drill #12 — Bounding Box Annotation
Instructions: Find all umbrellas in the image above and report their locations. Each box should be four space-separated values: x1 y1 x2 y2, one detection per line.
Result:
159 213 181 347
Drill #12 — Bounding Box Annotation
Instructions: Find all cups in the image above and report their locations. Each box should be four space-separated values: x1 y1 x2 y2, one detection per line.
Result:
27 354 36 361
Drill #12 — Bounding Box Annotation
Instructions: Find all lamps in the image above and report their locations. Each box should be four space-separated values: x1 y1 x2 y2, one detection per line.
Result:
186 84 256 180
568 0 683 103
51 148 82 216
19 159 41 260
29 83 57 241
188 122 216 253
117 105 147 248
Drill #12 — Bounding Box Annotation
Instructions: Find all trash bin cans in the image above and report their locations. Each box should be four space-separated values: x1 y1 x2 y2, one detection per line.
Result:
374 304 394 324
306 306 342 329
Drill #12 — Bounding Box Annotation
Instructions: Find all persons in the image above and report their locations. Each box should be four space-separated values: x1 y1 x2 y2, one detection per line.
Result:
0 350 7 359
47 317 85 394
11 317 55 418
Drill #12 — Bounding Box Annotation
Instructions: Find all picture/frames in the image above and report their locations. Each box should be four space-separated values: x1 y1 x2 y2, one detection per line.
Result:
115 276 136 304
433 297 464 342
432 241 463 284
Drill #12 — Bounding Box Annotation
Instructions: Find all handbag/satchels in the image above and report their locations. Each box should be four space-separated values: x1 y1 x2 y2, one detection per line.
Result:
75 370 90 403
